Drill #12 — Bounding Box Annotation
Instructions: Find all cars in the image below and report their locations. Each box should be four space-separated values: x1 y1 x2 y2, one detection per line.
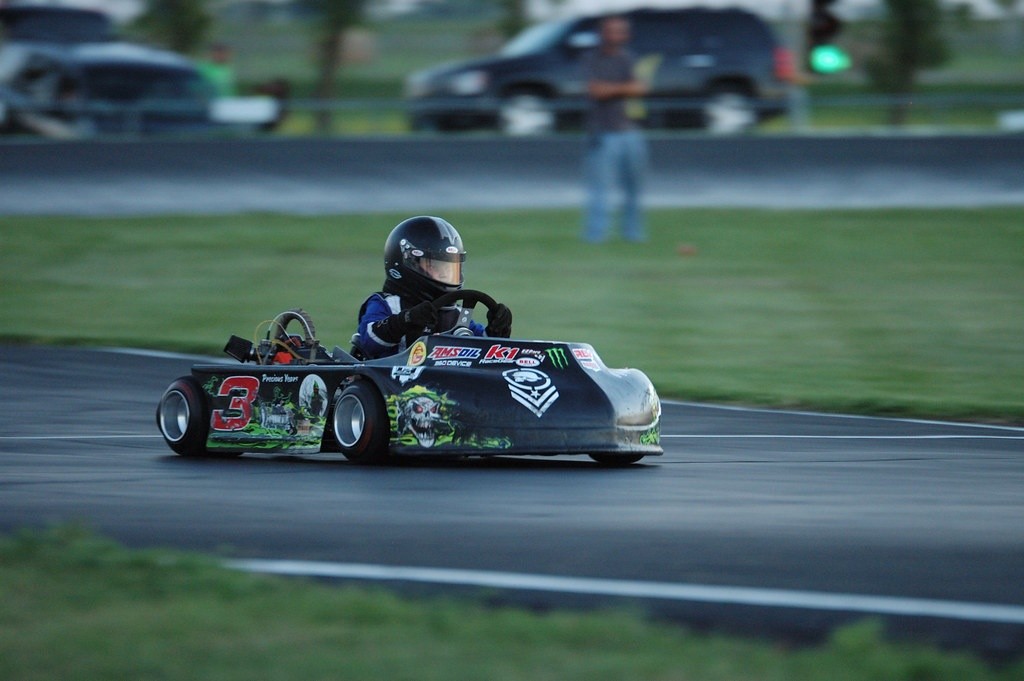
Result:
0 3 236 139
403 3 801 137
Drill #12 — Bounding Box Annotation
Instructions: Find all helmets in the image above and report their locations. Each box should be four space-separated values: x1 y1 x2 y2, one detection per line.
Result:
382 215 468 300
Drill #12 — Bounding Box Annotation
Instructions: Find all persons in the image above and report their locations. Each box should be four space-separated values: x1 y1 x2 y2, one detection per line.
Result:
582 18 651 243
349 215 513 361
198 43 240 95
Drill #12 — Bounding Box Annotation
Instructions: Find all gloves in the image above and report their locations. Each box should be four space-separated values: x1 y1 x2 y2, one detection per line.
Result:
405 300 438 328
485 303 513 338
